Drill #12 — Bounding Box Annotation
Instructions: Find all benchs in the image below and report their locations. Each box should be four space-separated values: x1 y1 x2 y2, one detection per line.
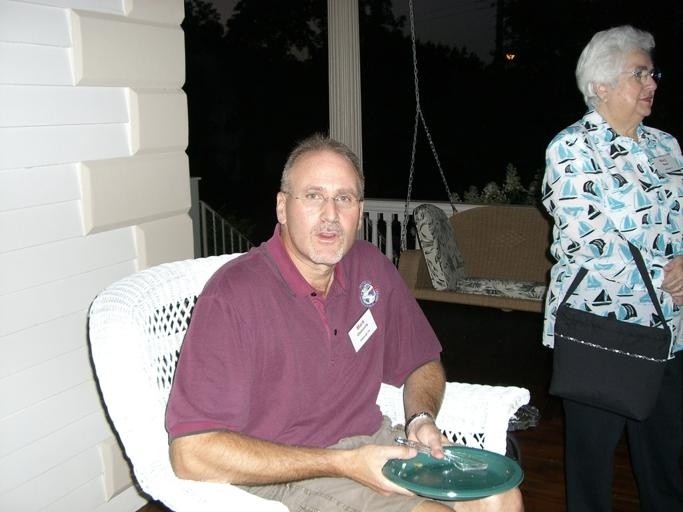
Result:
398 207 557 314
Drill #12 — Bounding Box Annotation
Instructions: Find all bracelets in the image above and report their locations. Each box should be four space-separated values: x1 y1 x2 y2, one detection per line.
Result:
405 411 435 441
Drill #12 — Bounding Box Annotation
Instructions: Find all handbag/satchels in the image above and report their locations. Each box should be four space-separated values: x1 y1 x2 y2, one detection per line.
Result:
548 238 674 421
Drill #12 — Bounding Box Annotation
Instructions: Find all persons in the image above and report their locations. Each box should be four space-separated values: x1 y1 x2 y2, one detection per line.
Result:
164 134 523 512
540 25 683 512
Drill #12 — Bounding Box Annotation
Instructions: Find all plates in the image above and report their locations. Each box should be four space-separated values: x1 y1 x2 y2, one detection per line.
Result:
382 446 524 501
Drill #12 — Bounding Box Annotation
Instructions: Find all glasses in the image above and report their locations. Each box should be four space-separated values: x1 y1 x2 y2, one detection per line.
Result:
621 69 662 83
283 189 364 211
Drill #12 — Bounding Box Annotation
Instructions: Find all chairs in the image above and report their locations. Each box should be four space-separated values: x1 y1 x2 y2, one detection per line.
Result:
88 253 540 512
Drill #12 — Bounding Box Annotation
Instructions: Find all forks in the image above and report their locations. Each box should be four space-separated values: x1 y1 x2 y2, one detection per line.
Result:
394 436 488 471
